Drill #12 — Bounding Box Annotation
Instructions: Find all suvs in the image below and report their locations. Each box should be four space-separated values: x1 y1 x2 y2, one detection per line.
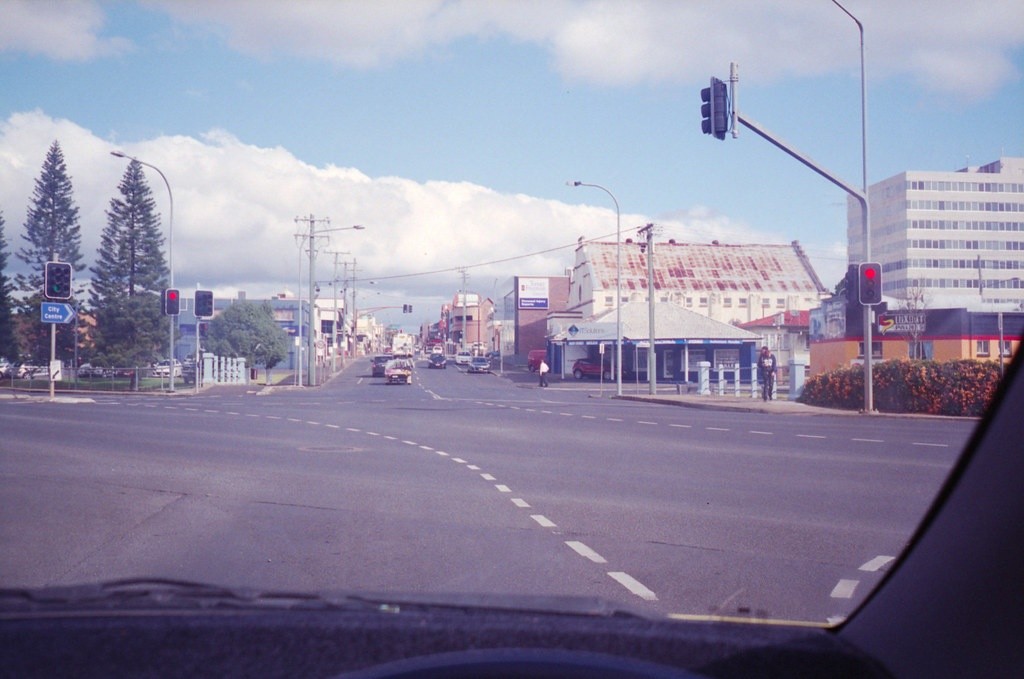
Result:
574 358 624 380
153 360 184 376
0 360 52 379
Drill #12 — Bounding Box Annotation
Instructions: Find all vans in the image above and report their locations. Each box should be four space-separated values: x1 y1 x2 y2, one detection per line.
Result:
528 350 547 373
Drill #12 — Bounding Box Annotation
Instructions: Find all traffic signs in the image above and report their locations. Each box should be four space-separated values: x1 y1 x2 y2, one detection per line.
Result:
40 302 76 323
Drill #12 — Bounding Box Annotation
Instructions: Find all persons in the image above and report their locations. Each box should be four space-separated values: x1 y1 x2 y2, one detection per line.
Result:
540 359 549 387
757 347 776 402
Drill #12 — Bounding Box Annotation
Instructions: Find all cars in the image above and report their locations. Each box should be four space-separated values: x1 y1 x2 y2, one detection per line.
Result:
468 357 491 373
455 350 474 364
76 367 136 377
370 346 416 384
424 346 448 368
487 351 499 359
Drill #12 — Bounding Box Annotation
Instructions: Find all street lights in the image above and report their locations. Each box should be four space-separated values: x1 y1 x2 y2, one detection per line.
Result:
110 150 174 393
297 225 364 388
573 181 627 397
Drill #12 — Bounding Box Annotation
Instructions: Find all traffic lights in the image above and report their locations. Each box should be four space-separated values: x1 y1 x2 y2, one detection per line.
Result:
701 77 729 140
844 263 884 306
45 261 73 299
403 304 412 313
163 289 180 315
194 290 213 316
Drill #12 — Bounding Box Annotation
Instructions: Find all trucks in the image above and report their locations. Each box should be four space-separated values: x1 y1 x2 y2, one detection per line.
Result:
391 333 416 357
